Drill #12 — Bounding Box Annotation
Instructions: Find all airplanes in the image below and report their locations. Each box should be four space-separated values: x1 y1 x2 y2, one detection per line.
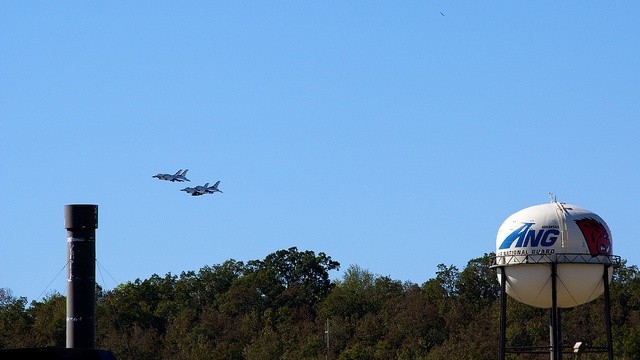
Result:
181 180 223 197
153 169 190 182
